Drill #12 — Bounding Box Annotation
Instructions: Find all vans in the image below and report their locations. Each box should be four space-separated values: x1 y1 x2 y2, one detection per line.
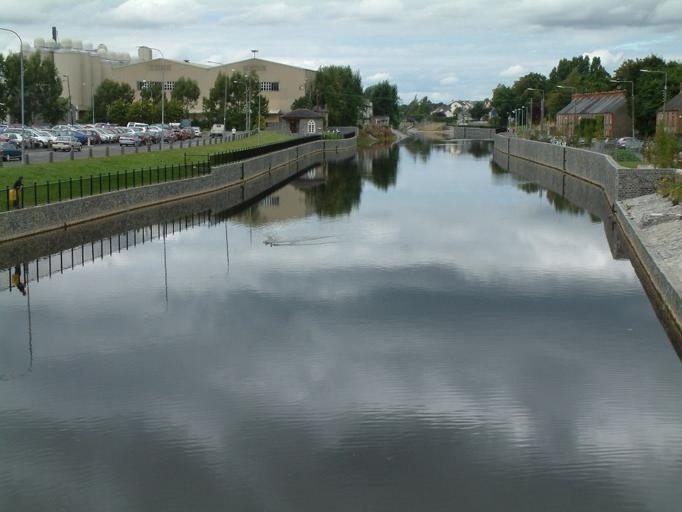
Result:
209 124 224 137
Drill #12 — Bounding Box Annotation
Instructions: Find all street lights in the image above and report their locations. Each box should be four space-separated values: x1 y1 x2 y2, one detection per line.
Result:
232 68 251 136
183 59 228 139
233 81 248 132
507 87 546 135
640 69 668 133
63 74 73 123
557 86 577 139
610 79 635 139
139 46 165 150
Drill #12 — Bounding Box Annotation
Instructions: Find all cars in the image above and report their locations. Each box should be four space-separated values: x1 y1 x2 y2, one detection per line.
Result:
0 121 203 162
537 134 644 150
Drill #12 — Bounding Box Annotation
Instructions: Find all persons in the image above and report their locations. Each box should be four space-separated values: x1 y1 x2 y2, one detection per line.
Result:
14 264 27 297
13 175 25 210
